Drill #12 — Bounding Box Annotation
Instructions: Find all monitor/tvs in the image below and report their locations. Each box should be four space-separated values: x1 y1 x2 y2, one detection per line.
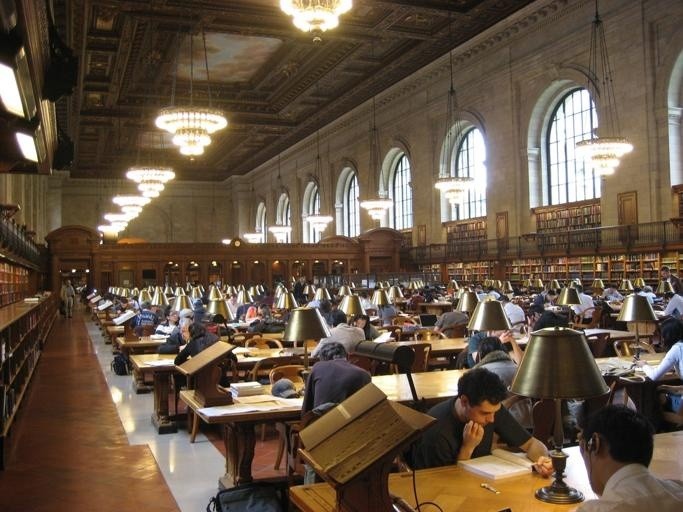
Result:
142 269 156 279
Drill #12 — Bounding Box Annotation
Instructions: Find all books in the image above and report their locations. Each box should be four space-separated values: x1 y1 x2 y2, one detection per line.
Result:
298 381 418 485
419 202 659 291
456 446 538 481
0 290 56 423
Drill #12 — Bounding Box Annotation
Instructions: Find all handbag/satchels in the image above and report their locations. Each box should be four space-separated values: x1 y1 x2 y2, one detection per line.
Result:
214 481 282 512
113 356 130 375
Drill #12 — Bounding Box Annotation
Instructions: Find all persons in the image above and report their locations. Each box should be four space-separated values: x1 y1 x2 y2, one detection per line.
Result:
411 366 556 479
84 266 683 430
60 279 77 320
572 402 683 512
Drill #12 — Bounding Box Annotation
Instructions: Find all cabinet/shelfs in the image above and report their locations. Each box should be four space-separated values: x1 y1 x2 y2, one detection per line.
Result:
420 199 683 290
0 247 56 470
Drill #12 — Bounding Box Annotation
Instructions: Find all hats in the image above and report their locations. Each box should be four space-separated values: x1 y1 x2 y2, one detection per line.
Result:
271 378 296 398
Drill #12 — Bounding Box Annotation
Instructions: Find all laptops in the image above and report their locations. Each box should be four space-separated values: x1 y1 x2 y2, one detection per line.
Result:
420 314 437 327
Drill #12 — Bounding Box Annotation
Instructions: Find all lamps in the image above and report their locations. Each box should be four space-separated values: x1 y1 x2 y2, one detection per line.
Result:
243 157 263 245
268 112 292 242
97 0 229 244
279 0 353 43
0 42 37 120
15 120 46 163
575 0 634 175
360 41 393 217
306 80 333 233
434 1 475 204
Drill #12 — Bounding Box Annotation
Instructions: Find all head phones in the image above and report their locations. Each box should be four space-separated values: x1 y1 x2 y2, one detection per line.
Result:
587 436 596 452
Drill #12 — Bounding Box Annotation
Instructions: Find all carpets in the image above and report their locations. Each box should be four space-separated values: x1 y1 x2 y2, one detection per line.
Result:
1 307 181 512
